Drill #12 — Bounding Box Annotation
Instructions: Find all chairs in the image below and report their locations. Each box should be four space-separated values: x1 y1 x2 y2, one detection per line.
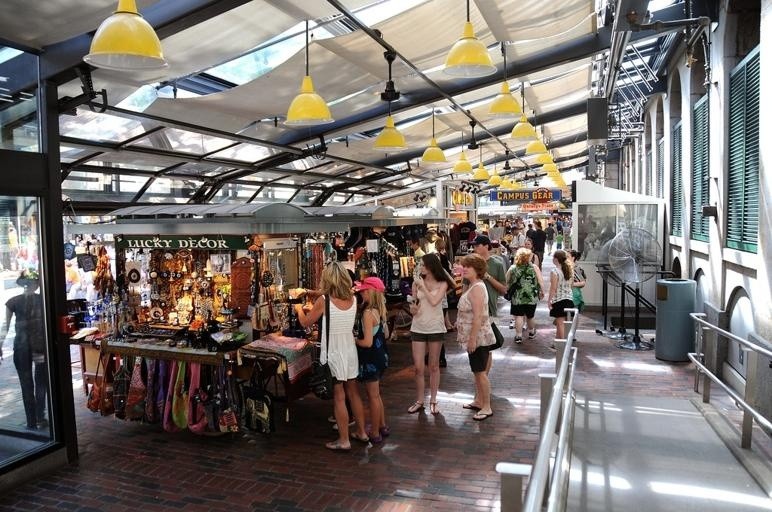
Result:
509 319 537 344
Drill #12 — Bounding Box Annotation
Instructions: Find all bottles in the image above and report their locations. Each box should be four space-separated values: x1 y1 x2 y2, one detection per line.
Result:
467 235 490 247
355 277 386 293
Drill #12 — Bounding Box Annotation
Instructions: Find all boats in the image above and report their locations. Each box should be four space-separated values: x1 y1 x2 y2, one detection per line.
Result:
587 97 609 140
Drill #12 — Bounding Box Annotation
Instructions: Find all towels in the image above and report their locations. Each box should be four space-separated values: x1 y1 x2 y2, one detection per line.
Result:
655 278 697 362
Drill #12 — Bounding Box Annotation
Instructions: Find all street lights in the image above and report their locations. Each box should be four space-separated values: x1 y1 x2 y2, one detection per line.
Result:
87 382 250 440
487 322 504 351
242 385 275 434
310 363 333 400
504 283 518 301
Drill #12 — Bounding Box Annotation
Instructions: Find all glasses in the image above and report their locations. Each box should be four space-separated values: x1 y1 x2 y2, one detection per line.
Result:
488 42 523 115
82 0 168 72
372 51 409 152
470 142 528 190
442 0 497 78
421 107 448 163
283 19 335 128
511 81 568 190
452 130 473 174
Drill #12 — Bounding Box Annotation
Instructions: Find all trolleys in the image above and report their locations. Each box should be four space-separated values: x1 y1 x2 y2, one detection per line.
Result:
598 239 634 340
608 228 664 351
354 49 436 112
468 119 489 149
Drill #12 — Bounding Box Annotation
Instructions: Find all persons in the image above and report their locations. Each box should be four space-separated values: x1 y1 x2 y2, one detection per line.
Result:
328 269 363 430
408 254 455 414
65 262 82 299
0 268 49 429
352 276 391 443
412 214 610 368
456 254 497 421
294 262 369 450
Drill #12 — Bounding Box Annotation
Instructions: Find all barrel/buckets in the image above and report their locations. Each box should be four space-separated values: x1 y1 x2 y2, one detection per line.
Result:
326 414 391 450
463 403 481 410
472 410 493 421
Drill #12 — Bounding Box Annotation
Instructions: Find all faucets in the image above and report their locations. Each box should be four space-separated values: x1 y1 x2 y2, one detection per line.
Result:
408 400 423 413
430 402 439 414
447 325 455 332
